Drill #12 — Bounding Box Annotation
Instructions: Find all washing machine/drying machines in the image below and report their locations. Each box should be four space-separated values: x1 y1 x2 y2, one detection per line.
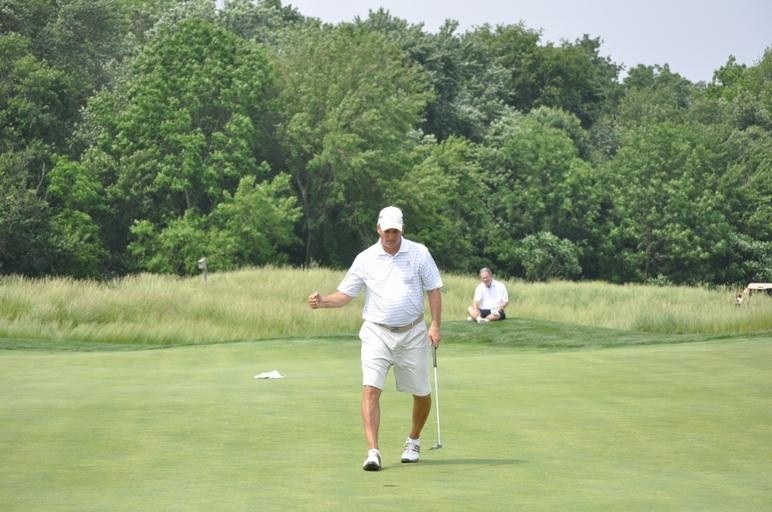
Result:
428 337 443 450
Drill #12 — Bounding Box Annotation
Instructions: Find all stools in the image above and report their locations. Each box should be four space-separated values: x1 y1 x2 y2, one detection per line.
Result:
363 314 424 333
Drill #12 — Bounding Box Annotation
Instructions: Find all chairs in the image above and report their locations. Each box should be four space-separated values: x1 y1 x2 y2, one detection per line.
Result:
400 439 420 462
363 449 381 472
477 318 489 324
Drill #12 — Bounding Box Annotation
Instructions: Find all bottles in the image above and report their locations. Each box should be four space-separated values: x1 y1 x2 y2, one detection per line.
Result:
377 206 403 233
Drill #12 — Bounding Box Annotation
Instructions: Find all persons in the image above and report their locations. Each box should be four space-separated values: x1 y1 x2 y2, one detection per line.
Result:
306 204 443 470
465 268 509 324
735 294 743 305
